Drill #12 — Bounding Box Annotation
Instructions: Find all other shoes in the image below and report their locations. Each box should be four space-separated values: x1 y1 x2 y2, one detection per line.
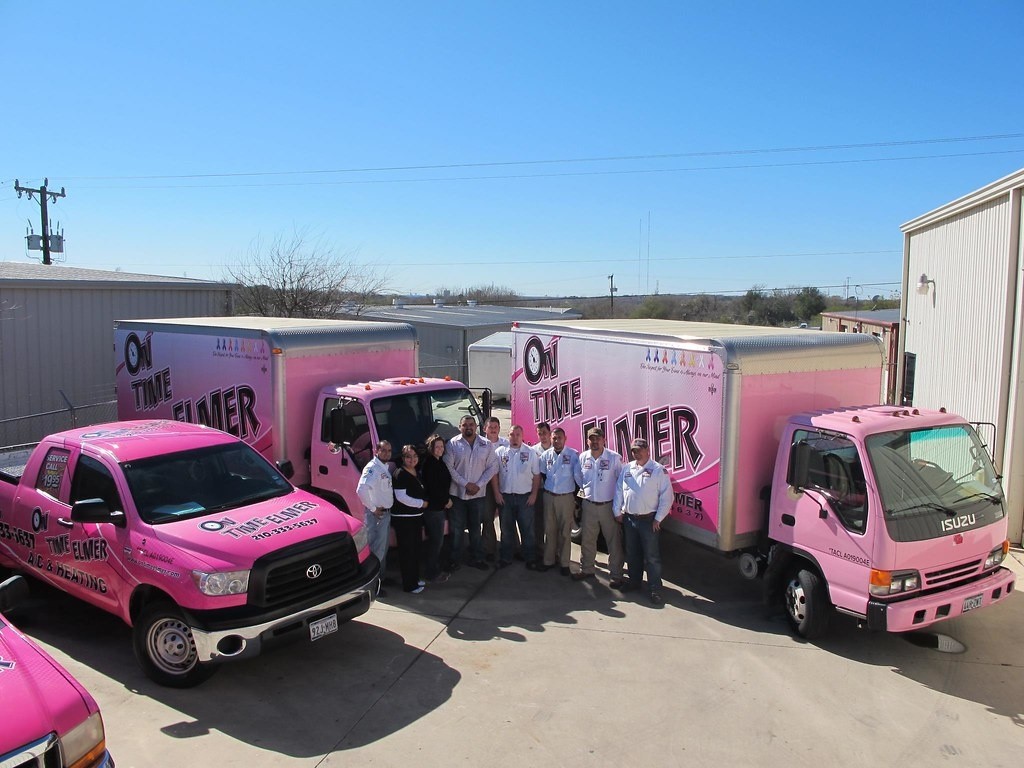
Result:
609 579 621 588
571 570 596 580
619 580 642 592
417 579 425 585
434 556 556 582
412 586 424 593
560 566 571 576
652 591 666 606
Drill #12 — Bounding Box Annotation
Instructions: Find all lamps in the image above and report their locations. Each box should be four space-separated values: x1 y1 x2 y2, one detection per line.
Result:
916 274 935 295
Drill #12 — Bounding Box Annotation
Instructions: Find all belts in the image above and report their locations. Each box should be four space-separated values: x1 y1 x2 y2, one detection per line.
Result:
545 490 571 497
503 493 531 496
624 512 655 519
382 509 389 512
586 498 613 505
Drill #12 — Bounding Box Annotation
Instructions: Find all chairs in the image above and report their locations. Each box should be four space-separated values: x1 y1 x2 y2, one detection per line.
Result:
824 454 854 492
186 459 223 494
387 396 417 425
344 411 357 437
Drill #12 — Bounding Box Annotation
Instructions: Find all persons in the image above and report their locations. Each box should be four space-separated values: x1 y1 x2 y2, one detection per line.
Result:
538 428 580 576
391 445 428 593
482 417 524 563
491 425 540 570
356 440 393 598
530 422 560 564
613 438 675 603
442 415 499 570
572 428 624 587
419 434 453 585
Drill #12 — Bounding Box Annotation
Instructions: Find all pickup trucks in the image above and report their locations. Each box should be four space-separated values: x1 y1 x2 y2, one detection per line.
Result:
0 419 384 690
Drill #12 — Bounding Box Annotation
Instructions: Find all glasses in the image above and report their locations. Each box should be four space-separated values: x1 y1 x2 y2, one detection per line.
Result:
405 454 419 458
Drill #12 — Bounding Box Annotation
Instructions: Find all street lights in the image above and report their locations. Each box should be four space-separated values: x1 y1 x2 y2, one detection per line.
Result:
608 274 618 316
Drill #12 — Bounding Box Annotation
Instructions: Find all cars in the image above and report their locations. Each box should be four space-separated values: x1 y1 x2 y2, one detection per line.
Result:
0 572 119 768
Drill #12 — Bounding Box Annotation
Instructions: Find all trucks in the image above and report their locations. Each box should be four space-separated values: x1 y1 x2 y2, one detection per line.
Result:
509 316 1017 643
112 315 494 551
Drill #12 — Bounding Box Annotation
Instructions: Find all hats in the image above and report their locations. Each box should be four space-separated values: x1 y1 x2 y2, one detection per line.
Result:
588 427 604 438
630 438 648 451
